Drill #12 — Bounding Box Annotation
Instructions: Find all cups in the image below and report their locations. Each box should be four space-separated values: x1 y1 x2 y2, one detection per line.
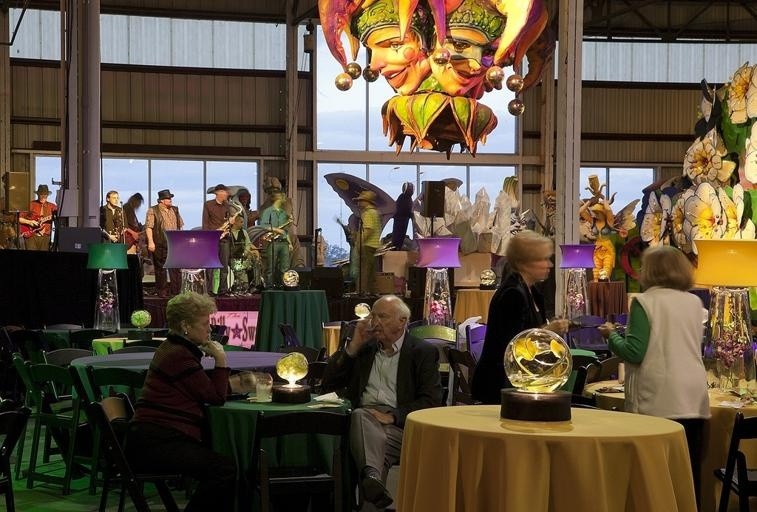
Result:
255 377 272 402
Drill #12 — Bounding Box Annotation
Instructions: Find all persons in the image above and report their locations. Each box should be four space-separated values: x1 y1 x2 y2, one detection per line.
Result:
349 1 433 96
227 214 261 295
100 190 126 243
319 293 444 512
122 192 145 250
471 228 572 404
430 0 508 97
233 189 260 228
348 190 381 296
145 189 184 296
122 292 237 511
259 189 294 291
599 244 712 512
201 184 235 296
259 176 305 269
18 184 58 251
578 194 617 282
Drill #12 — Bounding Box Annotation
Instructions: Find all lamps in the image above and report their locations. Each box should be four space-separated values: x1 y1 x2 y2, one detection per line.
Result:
160 227 228 300
86 242 135 332
692 238 757 386
413 236 463 332
556 243 598 320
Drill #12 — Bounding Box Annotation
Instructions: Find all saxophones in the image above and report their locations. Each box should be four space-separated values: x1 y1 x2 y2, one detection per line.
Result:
216 197 242 240
261 213 294 242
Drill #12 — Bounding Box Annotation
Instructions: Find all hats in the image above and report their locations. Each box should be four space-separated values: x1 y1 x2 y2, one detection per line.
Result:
34 185 51 195
215 184 230 191
262 177 282 193
157 190 174 200
352 191 377 205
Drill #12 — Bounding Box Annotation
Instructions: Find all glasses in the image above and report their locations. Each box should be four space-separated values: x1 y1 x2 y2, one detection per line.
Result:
266 190 279 194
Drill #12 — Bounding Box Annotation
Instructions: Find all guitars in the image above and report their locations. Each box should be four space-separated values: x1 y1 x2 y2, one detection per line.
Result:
124 227 147 250
18 215 53 239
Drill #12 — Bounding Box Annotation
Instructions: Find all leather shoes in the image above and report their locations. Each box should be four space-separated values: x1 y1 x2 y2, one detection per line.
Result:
361 475 393 508
